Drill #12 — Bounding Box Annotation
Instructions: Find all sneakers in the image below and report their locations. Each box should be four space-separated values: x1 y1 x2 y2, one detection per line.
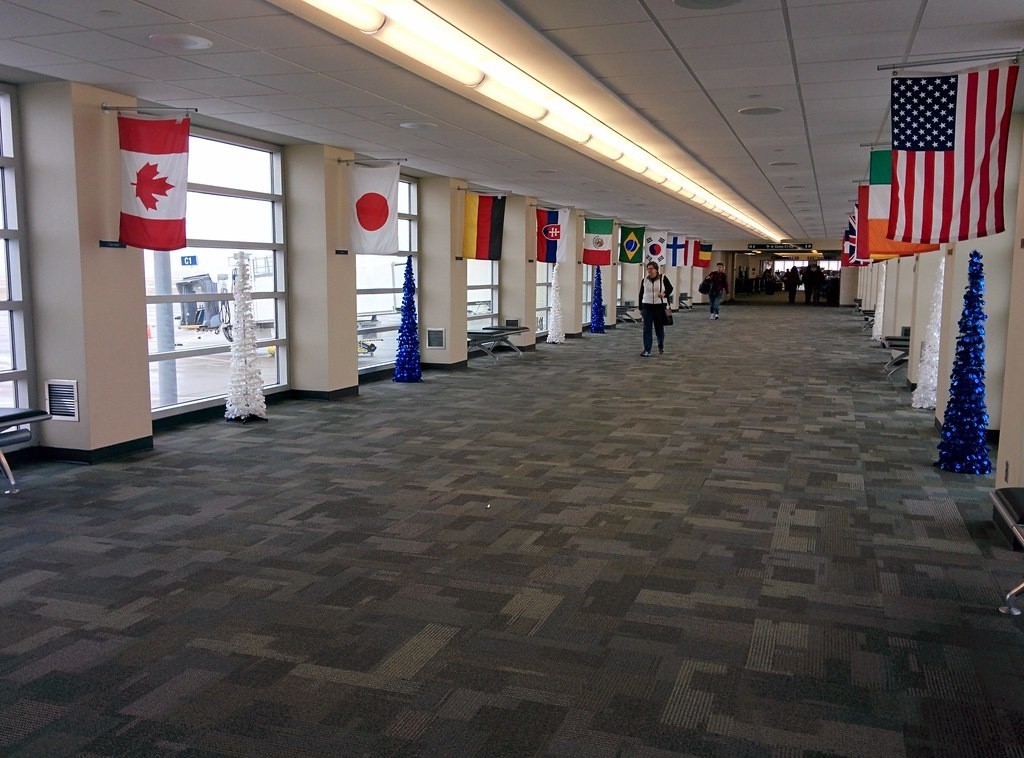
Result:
640 351 652 356
709 314 719 320
658 348 664 355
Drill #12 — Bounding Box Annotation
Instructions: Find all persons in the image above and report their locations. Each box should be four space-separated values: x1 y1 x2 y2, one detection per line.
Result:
705 263 729 319
752 264 824 305
638 261 673 356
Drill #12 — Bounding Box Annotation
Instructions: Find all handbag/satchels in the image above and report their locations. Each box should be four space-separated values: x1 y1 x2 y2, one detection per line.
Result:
699 279 713 294
663 307 673 325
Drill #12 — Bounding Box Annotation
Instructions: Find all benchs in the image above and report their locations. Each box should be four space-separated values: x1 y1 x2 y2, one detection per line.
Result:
0 408 52 493
861 310 876 331
854 298 862 314
882 336 910 381
466 325 529 365
668 296 693 312
988 487 1024 618
616 306 639 324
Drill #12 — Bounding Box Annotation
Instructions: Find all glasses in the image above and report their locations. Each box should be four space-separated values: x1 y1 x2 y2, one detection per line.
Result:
647 268 655 270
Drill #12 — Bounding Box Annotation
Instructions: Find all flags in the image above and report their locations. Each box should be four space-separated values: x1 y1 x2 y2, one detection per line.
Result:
583 219 612 265
352 167 398 255
534 206 571 265
841 147 940 268
619 227 714 268
116 112 188 251
464 191 505 262
888 63 1019 244
